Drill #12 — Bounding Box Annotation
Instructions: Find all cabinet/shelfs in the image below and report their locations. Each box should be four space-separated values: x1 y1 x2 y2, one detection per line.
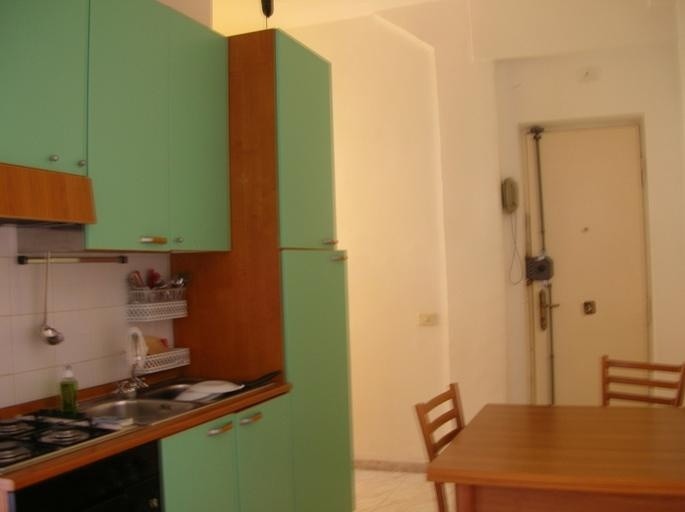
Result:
17 0 231 252
171 28 338 249
170 249 353 512
159 391 294 512
0 0 89 177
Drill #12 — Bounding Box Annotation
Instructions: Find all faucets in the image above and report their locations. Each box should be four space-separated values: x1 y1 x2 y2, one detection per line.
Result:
119 378 150 399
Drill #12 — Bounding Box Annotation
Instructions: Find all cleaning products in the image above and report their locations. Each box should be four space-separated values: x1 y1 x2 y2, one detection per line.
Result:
60 362 78 411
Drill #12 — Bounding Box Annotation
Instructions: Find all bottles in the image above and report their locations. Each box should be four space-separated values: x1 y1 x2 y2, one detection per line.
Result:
59 364 80 419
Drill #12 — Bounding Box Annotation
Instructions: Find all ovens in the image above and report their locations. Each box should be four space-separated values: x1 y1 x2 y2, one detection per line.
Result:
5 440 162 512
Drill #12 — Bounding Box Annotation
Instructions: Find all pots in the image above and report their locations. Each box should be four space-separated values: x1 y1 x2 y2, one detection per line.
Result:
214 368 280 402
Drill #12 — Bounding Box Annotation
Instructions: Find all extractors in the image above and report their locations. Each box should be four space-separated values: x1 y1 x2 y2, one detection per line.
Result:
0 162 97 231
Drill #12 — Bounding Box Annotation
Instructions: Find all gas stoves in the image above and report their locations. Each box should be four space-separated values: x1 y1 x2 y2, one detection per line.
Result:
0 410 138 476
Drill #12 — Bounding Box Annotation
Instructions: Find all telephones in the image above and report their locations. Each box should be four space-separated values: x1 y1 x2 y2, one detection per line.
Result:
503 178 518 213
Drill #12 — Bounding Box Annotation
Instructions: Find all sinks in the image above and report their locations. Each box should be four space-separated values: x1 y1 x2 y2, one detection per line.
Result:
87 399 209 429
146 384 190 398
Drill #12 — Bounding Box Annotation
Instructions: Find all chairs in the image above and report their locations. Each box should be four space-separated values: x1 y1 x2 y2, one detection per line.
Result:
600 354 685 408
415 383 466 512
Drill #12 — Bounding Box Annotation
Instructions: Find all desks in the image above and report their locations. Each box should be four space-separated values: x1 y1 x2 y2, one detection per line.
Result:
427 403 685 512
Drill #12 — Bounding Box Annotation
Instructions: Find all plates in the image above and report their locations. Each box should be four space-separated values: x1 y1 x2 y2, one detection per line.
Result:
175 379 245 406
